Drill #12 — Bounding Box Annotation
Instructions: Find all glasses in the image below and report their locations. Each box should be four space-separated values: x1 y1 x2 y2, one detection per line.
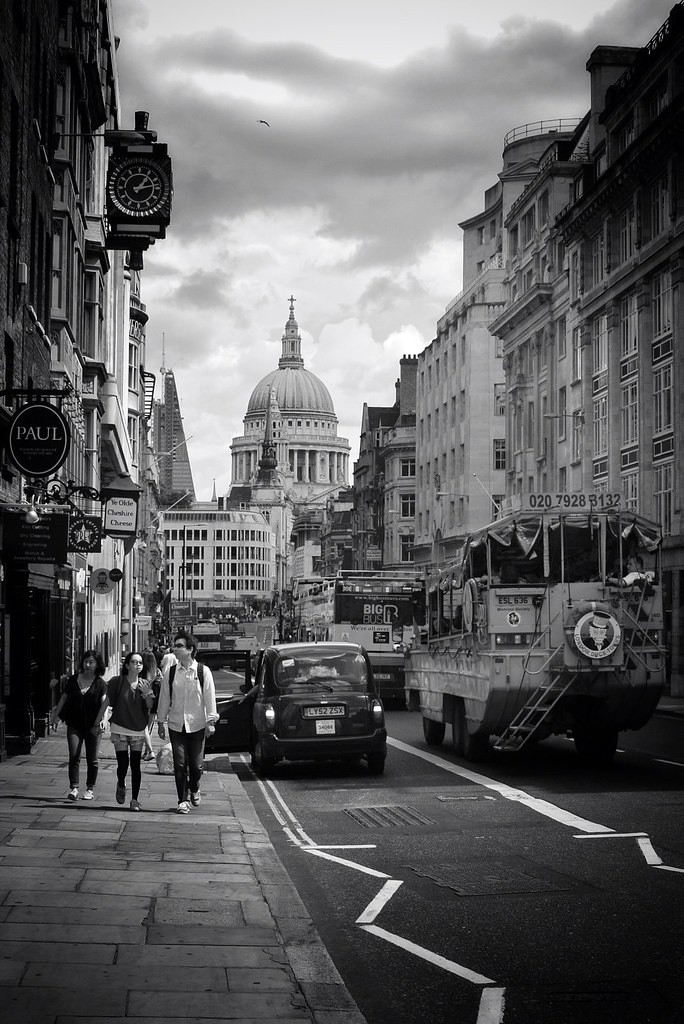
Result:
174 643 185 648
129 660 143 665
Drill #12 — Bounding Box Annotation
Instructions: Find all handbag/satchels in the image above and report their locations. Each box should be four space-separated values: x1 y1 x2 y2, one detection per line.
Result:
150 669 162 714
58 674 76 722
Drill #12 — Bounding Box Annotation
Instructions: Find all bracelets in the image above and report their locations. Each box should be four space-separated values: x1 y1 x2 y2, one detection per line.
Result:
93 724 100 727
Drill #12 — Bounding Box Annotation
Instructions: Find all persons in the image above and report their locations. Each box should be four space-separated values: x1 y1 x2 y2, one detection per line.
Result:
157 634 219 813
142 653 163 761
476 561 526 584
433 604 463 633
211 609 279 622
144 641 179 675
52 651 107 801
589 555 655 586
92 652 155 811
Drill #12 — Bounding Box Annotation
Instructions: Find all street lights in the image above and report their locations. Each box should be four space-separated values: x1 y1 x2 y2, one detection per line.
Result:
178 565 188 602
183 523 209 601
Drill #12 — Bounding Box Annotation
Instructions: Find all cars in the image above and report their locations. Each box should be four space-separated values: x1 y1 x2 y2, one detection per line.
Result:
254 642 388 776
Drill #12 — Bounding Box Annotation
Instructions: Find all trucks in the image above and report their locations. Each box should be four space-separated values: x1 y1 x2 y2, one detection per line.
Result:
396 505 667 767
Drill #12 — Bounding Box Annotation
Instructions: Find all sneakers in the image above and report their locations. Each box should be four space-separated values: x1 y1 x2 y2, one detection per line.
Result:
116 781 126 804
191 789 201 806
129 800 142 811
144 751 155 761
83 789 93 799
178 800 191 814
141 752 147 759
68 787 78 801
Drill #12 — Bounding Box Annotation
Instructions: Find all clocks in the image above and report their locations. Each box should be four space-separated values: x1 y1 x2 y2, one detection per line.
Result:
107 152 172 225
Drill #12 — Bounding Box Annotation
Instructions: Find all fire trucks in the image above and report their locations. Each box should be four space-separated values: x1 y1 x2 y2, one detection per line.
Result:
283 568 430 708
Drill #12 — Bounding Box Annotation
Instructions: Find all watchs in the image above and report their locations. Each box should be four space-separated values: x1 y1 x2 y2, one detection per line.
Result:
210 720 216 726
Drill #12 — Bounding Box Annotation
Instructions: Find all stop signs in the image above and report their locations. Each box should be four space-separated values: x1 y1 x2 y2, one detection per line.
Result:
109 568 122 582
